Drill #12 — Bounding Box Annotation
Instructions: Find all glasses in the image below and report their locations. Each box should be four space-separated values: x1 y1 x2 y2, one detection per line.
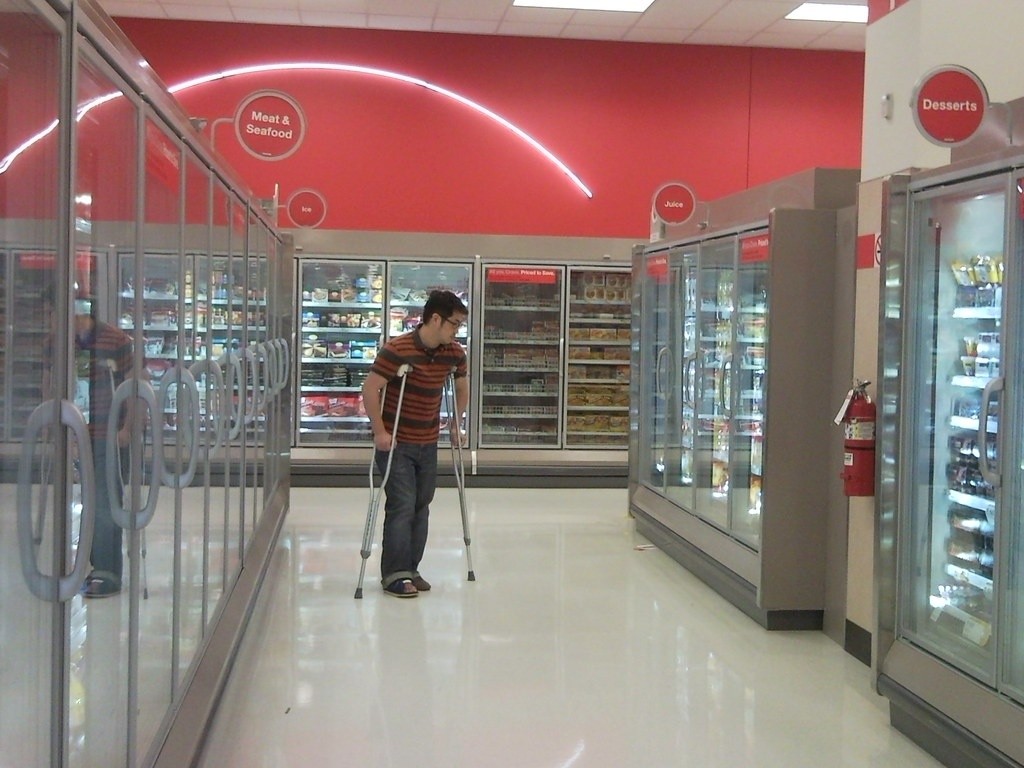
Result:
431 312 464 329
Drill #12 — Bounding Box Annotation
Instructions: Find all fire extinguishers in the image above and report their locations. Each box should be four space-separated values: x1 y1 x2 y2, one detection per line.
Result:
834 377 876 497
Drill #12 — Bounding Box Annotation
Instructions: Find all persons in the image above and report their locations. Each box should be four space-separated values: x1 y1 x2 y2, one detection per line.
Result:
362 290 469 598
41 284 152 598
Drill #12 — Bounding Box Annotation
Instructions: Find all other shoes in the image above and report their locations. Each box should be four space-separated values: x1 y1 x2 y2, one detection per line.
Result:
80 578 120 598
384 578 419 596
413 576 431 590
